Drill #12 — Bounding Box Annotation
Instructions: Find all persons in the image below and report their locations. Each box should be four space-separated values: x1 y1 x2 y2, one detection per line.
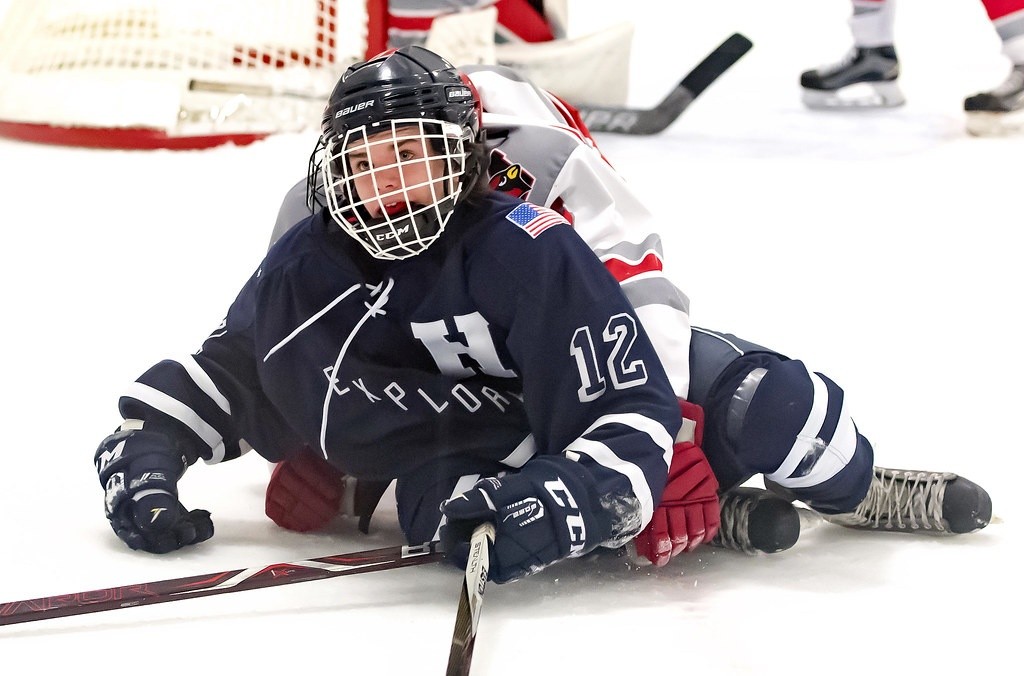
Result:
799 0 1024 137
388 0 555 56
92 45 1004 585
265 70 721 568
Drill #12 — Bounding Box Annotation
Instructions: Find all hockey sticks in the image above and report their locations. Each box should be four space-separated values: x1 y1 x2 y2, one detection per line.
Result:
183 33 754 136
0 535 446 625
447 519 496 676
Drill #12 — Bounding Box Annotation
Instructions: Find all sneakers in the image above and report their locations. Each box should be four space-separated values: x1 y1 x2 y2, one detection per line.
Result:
702 484 822 555
798 4 906 110
813 466 1004 536
962 10 1024 137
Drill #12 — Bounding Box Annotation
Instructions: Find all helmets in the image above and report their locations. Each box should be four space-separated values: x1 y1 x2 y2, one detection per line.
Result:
306 44 484 261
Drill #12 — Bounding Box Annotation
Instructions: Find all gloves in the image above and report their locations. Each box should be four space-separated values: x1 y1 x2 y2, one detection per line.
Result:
95 417 215 554
265 446 347 533
439 455 612 585
625 398 720 566
